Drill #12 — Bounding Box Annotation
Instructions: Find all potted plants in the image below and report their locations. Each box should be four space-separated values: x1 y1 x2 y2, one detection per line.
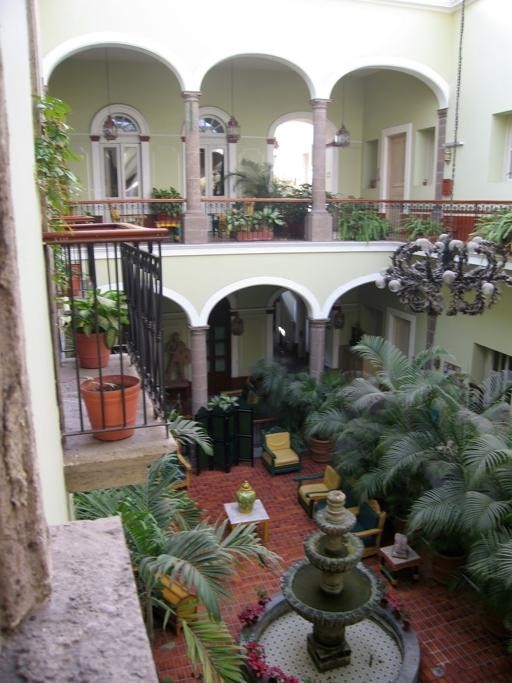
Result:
146 188 186 244
217 206 286 242
64 285 138 367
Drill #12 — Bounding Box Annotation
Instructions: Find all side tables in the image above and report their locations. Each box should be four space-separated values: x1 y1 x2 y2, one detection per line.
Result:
380 542 426 587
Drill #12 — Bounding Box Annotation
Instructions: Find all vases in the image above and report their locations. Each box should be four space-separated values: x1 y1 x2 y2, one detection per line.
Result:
76 374 143 443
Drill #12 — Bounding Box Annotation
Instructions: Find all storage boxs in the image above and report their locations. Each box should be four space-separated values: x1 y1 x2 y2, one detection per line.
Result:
142 568 205 637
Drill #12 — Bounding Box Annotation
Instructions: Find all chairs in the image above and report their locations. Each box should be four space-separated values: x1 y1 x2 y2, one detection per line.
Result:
257 429 387 563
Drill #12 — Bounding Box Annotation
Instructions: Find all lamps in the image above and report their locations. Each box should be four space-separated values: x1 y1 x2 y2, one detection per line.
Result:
224 59 245 144
100 45 120 141
335 78 352 147
373 0 512 321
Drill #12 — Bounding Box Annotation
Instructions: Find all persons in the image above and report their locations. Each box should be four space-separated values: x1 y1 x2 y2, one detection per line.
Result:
164 330 186 380
